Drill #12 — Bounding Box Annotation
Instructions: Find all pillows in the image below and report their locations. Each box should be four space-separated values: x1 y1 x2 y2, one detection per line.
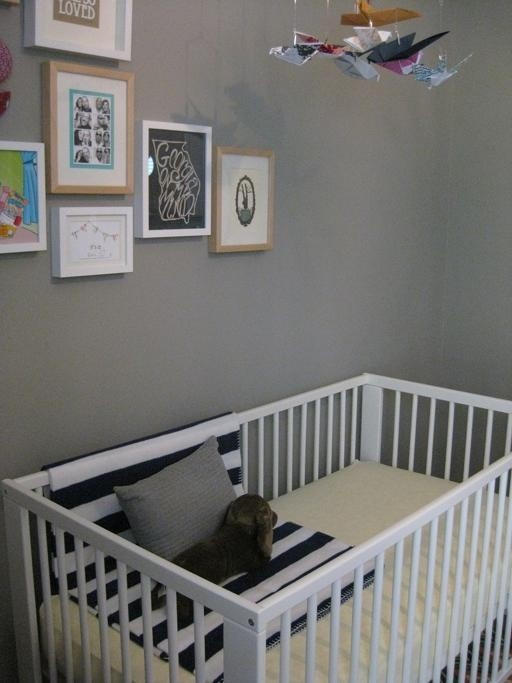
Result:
112 434 238 563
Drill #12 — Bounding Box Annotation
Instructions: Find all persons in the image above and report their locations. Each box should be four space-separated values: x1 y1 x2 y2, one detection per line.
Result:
75 96 110 164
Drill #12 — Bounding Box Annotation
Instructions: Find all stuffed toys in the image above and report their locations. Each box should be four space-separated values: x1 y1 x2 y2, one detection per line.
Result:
152 494 277 619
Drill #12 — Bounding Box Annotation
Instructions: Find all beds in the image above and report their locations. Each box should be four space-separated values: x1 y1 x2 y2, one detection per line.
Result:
2 369 512 682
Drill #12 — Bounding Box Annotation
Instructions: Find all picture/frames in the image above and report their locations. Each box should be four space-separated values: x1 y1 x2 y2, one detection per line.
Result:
51 206 133 277
23 1 133 61
1 140 46 254
43 60 133 194
135 120 212 238
208 147 278 253
